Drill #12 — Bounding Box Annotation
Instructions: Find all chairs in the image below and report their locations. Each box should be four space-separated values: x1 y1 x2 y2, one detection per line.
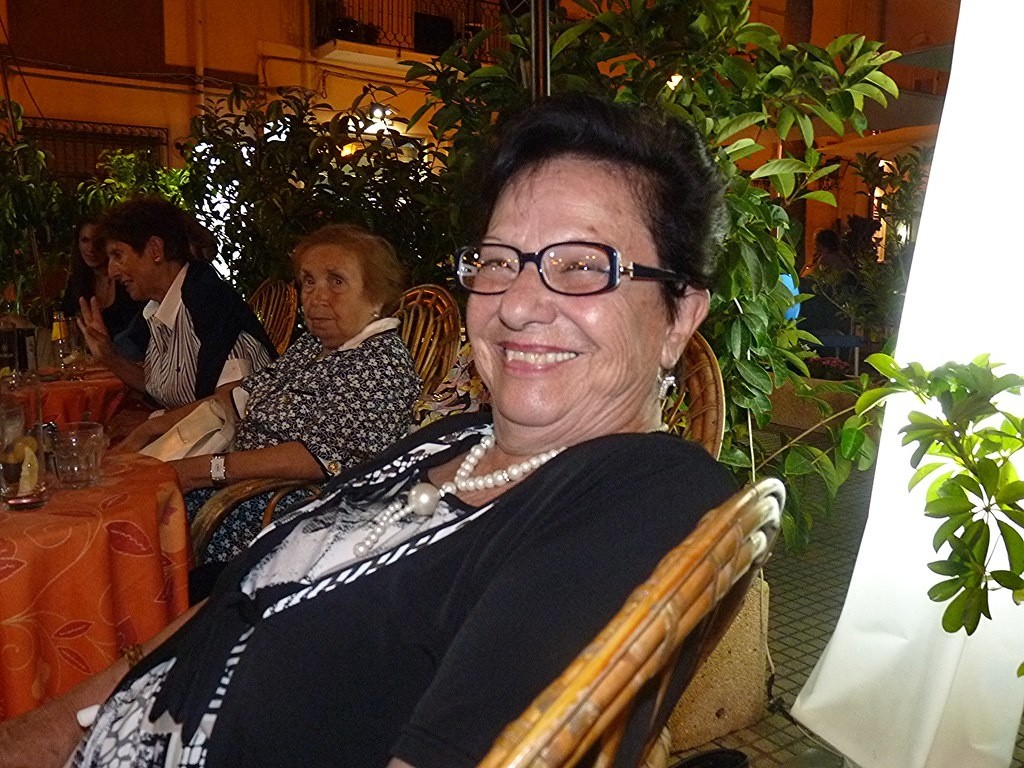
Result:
660 331 727 461
247 274 298 355
477 476 786 768
188 285 461 570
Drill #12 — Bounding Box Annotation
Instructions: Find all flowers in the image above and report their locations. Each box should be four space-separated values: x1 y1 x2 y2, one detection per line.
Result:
806 357 853 378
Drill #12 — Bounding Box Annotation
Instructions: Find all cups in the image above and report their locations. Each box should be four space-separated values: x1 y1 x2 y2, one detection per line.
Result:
49 423 103 490
0 374 46 510
59 317 86 372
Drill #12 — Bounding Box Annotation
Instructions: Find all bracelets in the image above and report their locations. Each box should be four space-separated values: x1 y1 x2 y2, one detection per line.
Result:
123 644 145 670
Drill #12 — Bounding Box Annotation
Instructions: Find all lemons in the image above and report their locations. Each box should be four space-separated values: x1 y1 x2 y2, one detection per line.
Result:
17 446 39 497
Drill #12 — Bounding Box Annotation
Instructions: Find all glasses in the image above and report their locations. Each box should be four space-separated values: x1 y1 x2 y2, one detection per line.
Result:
456 241 708 296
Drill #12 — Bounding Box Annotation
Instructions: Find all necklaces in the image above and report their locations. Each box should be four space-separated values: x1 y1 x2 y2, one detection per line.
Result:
354 428 570 557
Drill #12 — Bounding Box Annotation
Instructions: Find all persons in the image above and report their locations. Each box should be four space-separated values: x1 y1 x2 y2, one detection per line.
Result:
74 196 280 456
0 92 743 768
1 212 50 278
105 224 425 563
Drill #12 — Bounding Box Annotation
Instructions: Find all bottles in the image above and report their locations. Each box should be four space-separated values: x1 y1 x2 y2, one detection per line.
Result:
52 312 71 363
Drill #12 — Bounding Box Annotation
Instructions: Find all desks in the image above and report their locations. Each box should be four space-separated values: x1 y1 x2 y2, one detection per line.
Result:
0 362 142 436
0 450 188 722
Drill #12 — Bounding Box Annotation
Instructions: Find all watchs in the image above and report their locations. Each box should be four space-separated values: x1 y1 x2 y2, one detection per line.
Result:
209 452 230 489
